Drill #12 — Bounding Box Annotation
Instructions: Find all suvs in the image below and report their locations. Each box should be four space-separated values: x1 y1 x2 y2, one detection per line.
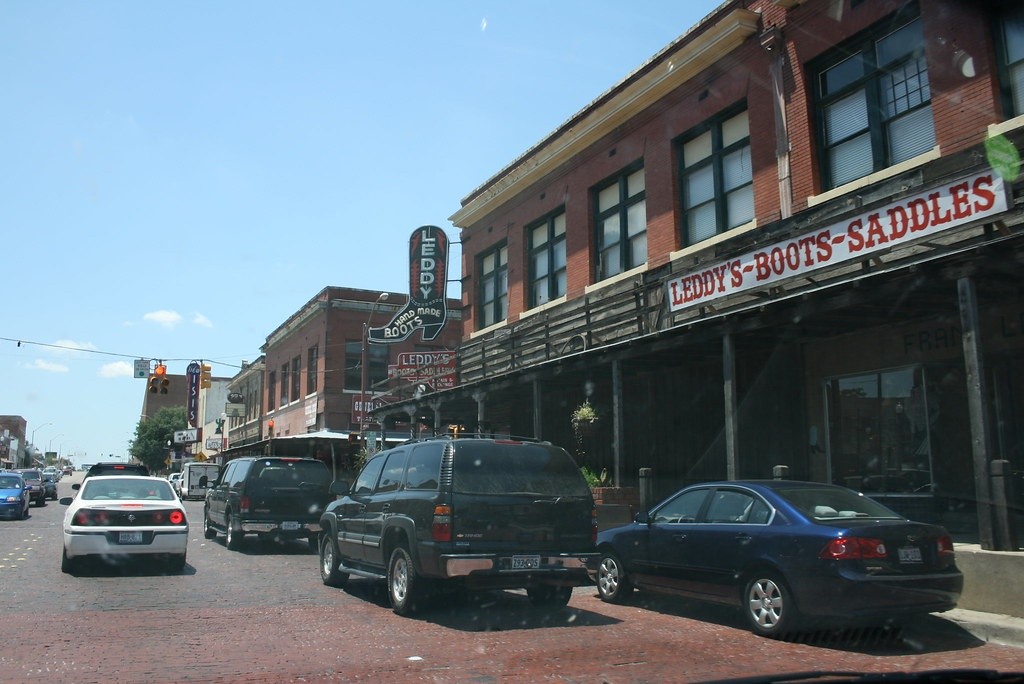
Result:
71 461 150 497
12 469 46 507
198 456 337 551
315 430 601 615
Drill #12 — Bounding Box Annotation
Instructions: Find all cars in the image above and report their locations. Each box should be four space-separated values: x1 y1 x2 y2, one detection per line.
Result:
58 474 191 574
32 465 73 498
590 478 964 639
0 473 33 521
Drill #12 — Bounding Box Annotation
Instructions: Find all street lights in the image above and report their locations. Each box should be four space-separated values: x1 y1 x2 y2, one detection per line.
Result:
49 433 64 464
355 292 389 467
30 422 53 467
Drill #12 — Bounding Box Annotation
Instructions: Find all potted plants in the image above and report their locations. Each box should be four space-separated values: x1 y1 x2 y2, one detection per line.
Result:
580 466 638 504
570 402 600 437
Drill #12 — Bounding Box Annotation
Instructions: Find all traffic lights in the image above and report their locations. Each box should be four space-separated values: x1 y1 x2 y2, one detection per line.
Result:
148 375 159 394
154 365 166 378
200 363 211 389
160 378 169 394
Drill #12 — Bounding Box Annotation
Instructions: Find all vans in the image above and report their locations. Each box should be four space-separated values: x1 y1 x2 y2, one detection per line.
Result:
168 461 222 499
